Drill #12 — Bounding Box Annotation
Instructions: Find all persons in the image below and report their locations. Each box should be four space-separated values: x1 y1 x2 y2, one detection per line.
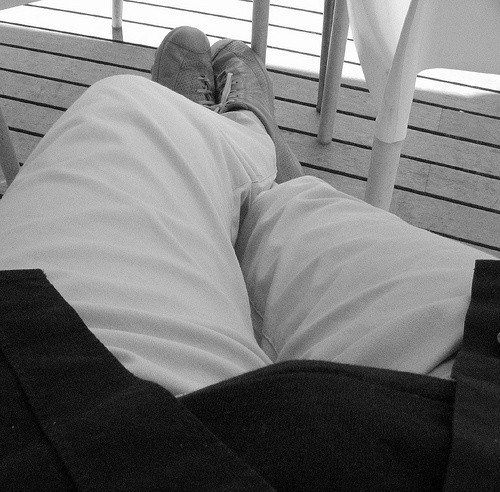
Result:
0 24 500 492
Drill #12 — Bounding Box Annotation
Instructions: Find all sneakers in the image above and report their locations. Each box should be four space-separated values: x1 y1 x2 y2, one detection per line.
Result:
210 39 303 184
151 26 216 110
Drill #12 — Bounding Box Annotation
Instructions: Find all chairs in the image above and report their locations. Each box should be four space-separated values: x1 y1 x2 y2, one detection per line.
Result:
314 0 500 214
